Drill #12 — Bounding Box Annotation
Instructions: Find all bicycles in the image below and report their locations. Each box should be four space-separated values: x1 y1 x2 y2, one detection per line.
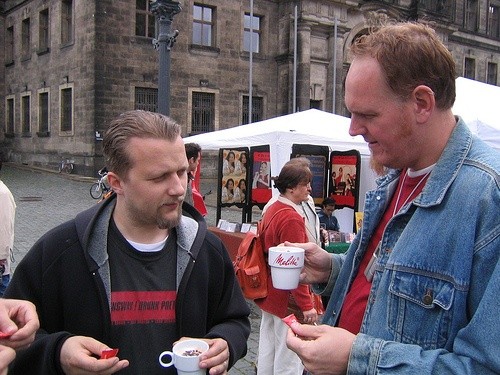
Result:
58 156 75 174
90 172 108 199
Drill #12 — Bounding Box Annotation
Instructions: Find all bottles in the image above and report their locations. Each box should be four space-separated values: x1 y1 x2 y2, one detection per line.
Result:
320 228 326 250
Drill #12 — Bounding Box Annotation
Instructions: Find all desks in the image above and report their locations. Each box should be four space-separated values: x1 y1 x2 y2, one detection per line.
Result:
208 227 247 262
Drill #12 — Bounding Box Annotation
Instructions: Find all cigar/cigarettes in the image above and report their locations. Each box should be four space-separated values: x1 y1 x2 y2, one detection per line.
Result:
313 322 317 327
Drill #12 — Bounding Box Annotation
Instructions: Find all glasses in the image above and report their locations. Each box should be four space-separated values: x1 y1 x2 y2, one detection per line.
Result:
326 206 333 211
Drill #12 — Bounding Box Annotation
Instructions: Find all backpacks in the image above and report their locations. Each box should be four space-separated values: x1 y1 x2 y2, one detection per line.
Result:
233 207 295 299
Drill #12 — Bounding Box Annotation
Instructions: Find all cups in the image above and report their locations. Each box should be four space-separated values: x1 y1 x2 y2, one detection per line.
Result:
159 339 209 375
268 247 305 290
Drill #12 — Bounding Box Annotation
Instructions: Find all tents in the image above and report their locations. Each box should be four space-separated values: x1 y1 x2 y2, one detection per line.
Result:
183 77 377 240
451 76 500 149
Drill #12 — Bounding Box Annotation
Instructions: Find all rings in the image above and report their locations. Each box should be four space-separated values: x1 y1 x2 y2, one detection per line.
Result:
308 318 311 322
224 361 228 368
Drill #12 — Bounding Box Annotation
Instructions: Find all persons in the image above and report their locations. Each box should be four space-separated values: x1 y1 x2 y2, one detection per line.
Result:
257 157 342 375
184 143 201 207
98 166 110 192
286 11 500 375
0 176 40 375
3 109 250 375
220 149 355 202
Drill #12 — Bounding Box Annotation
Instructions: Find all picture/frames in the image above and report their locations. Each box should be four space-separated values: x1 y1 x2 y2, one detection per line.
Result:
292 145 329 206
219 147 248 207
248 146 273 207
328 150 360 209
